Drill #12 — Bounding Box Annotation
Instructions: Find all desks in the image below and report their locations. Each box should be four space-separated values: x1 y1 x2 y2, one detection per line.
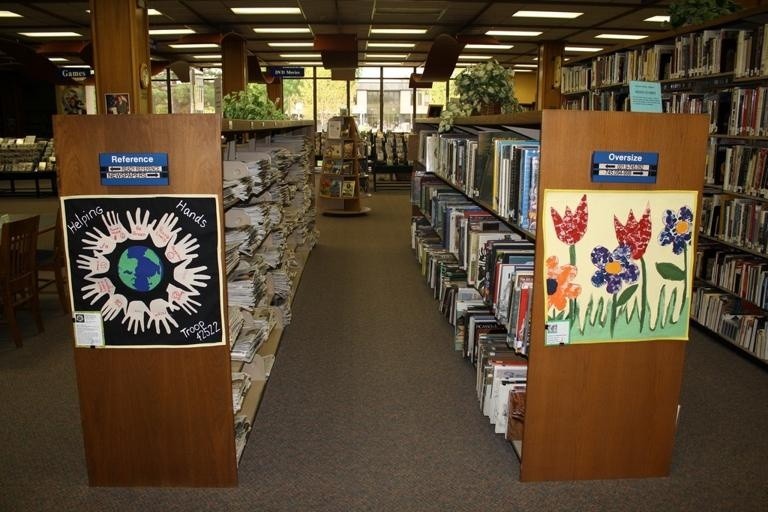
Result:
1 214 56 303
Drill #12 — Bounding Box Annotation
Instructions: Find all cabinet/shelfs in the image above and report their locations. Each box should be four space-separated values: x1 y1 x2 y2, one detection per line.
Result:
52 114 317 487
558 10 766 363
321 113 371 215
0 137 59 199
314 132 412 189
410 115 710 482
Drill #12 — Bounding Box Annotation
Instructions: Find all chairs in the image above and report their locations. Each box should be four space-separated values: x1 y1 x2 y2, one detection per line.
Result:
20 206 69 314
2 215 45 347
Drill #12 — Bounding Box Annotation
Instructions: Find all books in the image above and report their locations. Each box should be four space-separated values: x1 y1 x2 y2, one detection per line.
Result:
224 126 317 465
0 135 56 174
690 240 767 361
417 127 540 230
411 216 467 316
698 134 768 254
462 308 528 441
443 206 535 356
558 20 768 137
318 129 413 195
